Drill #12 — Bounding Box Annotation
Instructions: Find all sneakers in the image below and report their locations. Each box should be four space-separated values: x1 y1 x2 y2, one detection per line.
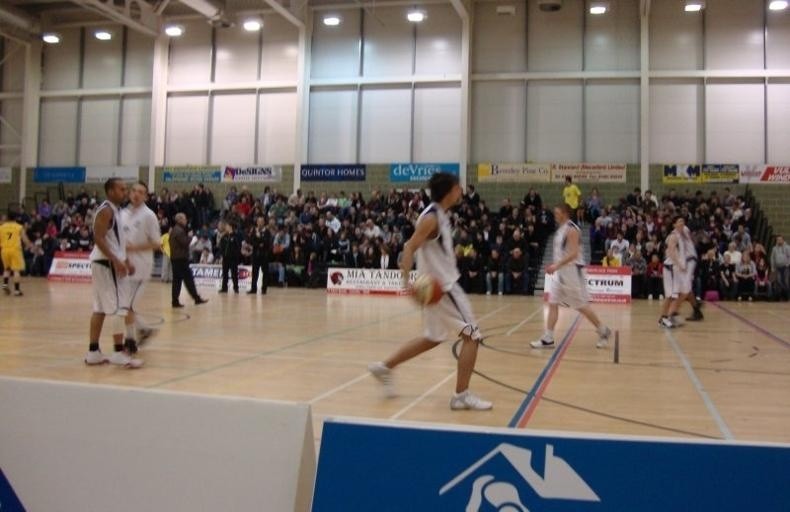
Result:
530 336 554 347
659 316 675 327
85 350 144 367
450 389 493 409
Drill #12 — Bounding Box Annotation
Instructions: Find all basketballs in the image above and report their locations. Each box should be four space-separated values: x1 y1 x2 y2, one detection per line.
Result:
411 272 444 306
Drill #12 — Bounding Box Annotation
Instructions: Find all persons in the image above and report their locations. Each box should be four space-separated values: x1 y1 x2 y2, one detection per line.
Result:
370 173 494 412
86 177 160 369
658 216 715 329
169 213 208 306
2 173 787 297
530 202 612 347
